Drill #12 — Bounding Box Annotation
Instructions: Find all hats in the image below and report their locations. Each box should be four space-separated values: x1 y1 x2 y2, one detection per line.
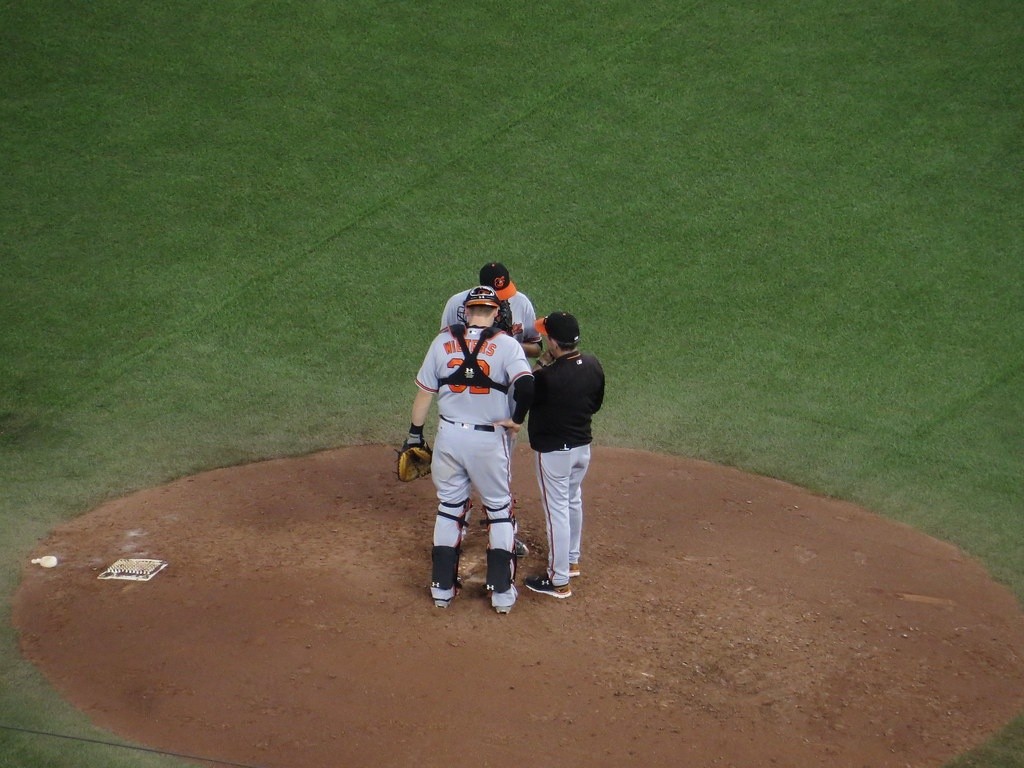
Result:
480 263 516 300
465 285 501 306
535 312 580 343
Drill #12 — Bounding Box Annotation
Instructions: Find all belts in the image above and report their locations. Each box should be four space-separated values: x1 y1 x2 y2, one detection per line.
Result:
439 415 508 432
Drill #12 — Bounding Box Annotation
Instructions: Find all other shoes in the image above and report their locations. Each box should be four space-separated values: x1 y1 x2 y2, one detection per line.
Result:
514 539 529 557
435 587 459 608
488 591 511 614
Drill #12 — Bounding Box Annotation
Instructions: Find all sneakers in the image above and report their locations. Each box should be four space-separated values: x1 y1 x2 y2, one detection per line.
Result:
568 563 580 577
525 576 572 598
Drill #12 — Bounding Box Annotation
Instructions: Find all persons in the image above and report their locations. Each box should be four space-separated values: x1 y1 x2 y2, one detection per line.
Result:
512 311 606 598
440 262 544 557
396 285 536 614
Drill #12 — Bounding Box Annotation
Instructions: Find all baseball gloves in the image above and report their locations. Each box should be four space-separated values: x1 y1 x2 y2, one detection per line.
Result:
395 437 434 484
